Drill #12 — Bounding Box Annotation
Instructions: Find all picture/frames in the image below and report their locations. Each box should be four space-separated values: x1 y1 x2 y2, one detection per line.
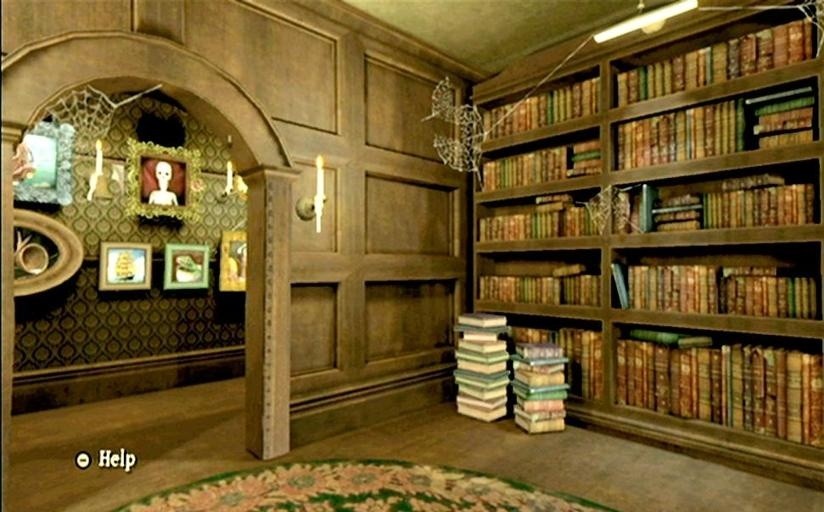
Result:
92 231 255 292
127 136 204 222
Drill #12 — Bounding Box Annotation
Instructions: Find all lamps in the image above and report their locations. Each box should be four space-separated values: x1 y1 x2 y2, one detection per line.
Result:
593 0 697 44
87 140 103 201
296 155 327 233
215 162 234 204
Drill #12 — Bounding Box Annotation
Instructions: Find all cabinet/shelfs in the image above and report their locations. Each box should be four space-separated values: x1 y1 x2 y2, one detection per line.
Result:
472 0 824 493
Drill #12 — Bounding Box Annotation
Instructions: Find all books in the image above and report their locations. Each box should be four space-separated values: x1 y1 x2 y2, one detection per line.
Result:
452 15 823 449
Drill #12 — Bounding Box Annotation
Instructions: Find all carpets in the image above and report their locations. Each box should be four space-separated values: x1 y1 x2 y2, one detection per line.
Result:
111 458 619 512
